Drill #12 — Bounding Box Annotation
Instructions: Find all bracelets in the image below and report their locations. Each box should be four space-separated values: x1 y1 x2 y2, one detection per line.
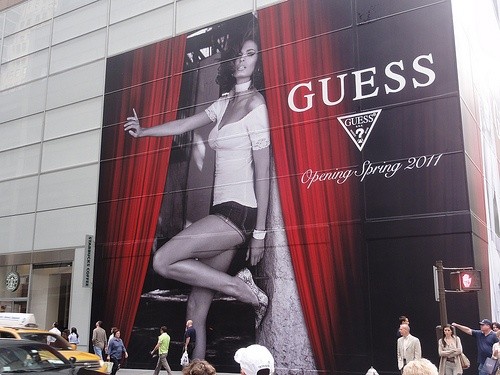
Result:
252 229 267 239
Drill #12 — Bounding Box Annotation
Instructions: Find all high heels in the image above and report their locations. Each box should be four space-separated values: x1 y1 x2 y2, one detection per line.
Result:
238 266 268 328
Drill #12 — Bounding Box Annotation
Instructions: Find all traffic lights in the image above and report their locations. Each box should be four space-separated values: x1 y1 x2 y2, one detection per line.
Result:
458 270 483 290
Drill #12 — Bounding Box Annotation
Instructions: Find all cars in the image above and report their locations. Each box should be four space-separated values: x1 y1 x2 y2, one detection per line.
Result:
0 313 109 374
0 338 109 375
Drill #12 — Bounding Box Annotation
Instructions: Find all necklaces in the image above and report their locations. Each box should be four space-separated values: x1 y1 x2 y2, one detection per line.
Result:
233 80 252 92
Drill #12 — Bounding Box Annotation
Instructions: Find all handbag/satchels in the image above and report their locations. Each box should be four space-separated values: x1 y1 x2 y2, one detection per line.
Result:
481 358 499 375
102 359 114 373
459 353 470 369
180 350 189 367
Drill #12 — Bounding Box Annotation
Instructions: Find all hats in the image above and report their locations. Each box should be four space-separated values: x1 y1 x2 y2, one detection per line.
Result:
479 319 491 326
234 344 274 375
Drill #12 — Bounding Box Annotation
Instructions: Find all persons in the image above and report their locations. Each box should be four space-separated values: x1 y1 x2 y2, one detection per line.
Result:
0 306 5 312
46 322 61 347
91 320 107 360
438 324 463 375
184 320 197 362
62 329 70 341
106 329 128 375
68 327 79 348
123 30 271 364
184 358 216 375
397 324 421 375
402 358 438 375
150 326 173 375
234 344 274 375
452 319 500 375
397 314 409 339
105 327 120 371
